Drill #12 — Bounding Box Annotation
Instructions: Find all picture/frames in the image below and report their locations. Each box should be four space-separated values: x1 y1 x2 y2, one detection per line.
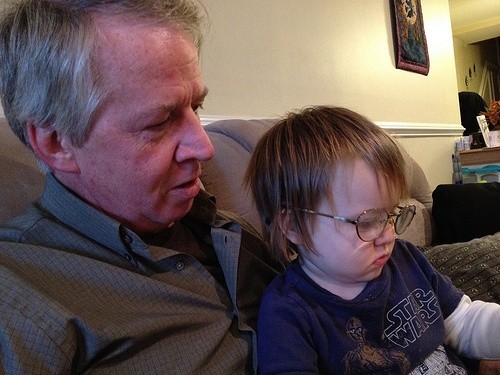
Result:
389 0 430 76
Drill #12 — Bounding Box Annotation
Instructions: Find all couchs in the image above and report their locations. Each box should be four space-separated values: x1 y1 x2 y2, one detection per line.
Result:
0 119 500 375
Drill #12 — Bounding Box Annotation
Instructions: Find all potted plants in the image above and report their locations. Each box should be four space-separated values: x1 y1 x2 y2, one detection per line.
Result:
479 99 500 148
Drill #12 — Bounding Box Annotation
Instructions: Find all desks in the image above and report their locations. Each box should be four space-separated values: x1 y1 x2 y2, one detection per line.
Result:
459 146 500 182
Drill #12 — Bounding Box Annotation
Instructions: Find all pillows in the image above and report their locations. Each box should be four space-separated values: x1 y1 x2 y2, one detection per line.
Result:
419 231 500 303
430 181 500 247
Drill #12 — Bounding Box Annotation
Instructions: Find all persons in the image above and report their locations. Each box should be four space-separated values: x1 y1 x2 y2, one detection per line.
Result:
1 0 292 375
239 102 500 375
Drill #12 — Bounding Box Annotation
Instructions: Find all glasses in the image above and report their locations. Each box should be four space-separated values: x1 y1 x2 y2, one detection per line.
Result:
284 200 416 243
